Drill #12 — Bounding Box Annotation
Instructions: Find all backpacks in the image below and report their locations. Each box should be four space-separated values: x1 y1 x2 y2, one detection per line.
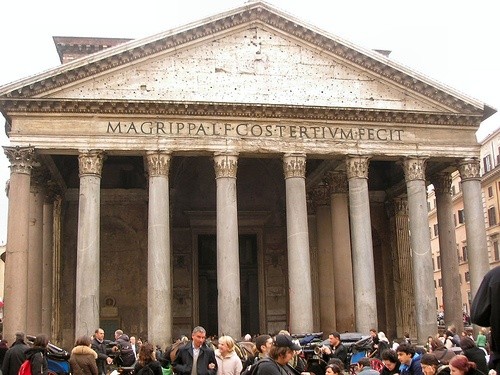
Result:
18 360 31 375
137 363 154 375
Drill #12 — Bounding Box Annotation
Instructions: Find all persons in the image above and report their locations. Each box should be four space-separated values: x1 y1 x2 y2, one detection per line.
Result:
0 327 500 375
463 312 471 327
470 266 500 375
436 305 445 337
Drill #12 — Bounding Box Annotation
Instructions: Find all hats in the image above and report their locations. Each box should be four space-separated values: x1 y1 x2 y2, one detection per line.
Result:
273 334 301 350
